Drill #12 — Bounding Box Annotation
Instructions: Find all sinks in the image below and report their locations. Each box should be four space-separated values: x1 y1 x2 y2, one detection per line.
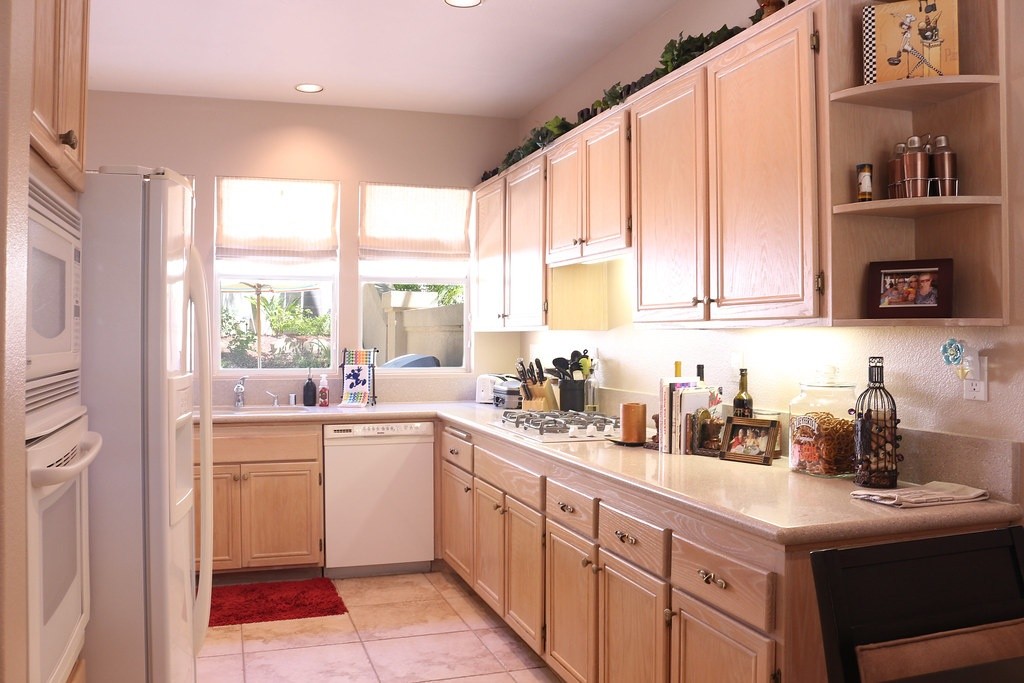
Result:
212 406 240 416
239 405 312 415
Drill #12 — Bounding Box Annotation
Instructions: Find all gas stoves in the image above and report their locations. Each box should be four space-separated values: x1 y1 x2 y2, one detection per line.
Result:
488 408 657 443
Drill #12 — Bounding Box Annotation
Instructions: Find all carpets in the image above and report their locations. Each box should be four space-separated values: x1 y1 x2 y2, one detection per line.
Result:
195 576 349 627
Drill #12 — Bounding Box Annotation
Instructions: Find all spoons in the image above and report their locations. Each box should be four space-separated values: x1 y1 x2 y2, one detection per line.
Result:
545 349 592 379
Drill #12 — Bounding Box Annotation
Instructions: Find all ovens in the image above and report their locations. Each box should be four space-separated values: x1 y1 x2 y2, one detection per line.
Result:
23 178 102 681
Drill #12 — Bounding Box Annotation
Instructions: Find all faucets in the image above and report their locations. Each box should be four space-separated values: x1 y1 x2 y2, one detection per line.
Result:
232 375 250 407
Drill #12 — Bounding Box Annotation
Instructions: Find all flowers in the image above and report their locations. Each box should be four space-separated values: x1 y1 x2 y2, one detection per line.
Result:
941 338 970 379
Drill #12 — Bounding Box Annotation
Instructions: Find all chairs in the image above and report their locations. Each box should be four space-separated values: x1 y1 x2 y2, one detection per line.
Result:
808 525 1024 683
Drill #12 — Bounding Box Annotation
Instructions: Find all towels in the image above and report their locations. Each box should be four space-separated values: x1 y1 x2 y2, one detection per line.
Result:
336 347 374 408
848 478 992 509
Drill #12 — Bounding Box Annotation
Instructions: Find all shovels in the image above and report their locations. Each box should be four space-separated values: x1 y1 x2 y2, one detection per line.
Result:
546 368 561 380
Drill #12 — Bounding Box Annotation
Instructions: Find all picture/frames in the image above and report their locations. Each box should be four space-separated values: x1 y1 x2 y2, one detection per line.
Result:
719 416 780 466
692 418 725 458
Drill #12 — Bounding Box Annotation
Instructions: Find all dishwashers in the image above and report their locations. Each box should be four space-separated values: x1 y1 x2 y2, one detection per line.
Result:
323 420 434 578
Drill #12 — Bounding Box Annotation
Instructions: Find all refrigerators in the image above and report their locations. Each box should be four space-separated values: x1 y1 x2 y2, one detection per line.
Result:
83 163 216 683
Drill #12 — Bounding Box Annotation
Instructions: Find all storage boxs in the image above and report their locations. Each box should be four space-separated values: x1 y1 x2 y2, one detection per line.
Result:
866 258 954 319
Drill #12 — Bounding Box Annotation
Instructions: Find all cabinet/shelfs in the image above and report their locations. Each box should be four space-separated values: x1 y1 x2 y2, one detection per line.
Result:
29 0 91 208
822 0 1014 327
471 147 608 332
546 97 630 268
441 419 473 590
192 421 324 585
630 0 827 332
663 501 1012 683
544 457 670 683
473 431 545 661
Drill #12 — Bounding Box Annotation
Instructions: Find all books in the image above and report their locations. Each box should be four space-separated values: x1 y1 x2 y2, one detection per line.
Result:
658 377 722 454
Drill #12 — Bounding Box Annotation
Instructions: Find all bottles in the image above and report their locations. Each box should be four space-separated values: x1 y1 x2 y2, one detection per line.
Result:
696 364 705 381
584 368 600 413
788 382 858 478
733 368 753 418
303 373 317 406
674 361 681 377
887 135 957 197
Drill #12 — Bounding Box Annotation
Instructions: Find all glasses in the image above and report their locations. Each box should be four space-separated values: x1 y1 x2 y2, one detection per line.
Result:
910 280 918 283
898 281 904 283
920 279 931 283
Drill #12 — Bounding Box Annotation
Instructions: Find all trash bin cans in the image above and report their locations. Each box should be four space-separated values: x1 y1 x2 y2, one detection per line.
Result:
378 354 440 368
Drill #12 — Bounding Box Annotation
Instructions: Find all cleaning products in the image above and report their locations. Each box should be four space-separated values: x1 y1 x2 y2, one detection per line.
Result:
302 366 317 407
317 372 332 408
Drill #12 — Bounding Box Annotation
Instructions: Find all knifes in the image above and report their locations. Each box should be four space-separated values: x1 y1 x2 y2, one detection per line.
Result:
515 357 544 401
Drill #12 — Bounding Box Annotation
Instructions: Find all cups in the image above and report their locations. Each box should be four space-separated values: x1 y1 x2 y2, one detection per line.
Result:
753 410 782 458
560 379 584 411
619 402 647 442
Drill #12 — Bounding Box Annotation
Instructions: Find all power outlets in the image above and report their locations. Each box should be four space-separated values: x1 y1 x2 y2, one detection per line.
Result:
963 356 988 402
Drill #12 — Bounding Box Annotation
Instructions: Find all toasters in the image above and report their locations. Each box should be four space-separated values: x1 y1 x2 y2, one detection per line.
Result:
475 374 517 404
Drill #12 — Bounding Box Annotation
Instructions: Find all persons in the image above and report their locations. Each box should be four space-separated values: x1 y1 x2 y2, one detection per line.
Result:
882 273 938 305
728 429 767 454
